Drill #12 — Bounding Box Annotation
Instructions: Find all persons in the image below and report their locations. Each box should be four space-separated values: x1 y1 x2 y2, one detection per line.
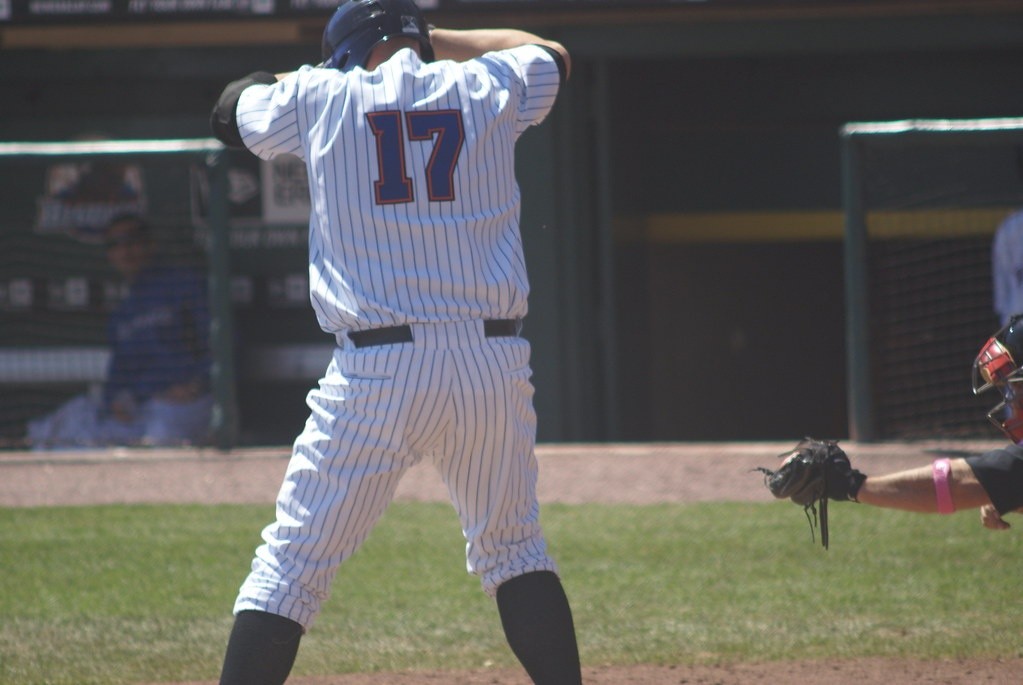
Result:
26 214 208 448
209 0 582 685
992 213 1023 446
765 315 1023 530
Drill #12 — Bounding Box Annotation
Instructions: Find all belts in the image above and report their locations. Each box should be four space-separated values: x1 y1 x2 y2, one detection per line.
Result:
348 319 518 348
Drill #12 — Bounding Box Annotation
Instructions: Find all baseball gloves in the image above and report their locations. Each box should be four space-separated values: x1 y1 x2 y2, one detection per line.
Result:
765 435 868 507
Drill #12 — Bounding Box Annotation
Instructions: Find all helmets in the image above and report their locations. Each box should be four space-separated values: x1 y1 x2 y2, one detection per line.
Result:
971 311 1023 444
321 0 436 72
